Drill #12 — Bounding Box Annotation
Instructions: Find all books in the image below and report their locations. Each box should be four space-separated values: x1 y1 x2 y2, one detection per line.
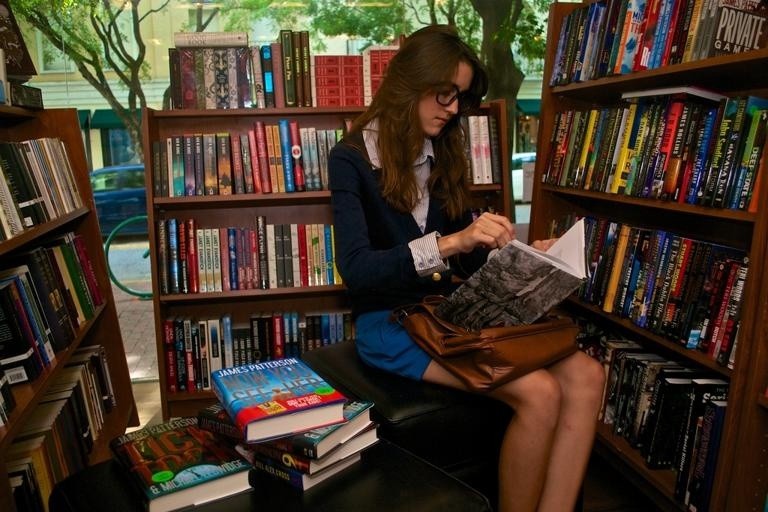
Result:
542 1 768 215
548 211 749 512
432 216 594 330
108 357 380 512
151 30 408 396
460 114 502 220
1 0 114 511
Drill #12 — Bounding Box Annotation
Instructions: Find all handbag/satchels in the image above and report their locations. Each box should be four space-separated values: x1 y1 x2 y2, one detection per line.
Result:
388 295 578 393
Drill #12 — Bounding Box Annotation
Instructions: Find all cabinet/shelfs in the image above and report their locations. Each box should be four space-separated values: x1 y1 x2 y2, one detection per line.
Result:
138 99 513 422
516 3 768 512
0 104 140 512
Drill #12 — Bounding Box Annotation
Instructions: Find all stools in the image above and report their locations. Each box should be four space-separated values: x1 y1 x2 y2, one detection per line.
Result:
305 338 512 491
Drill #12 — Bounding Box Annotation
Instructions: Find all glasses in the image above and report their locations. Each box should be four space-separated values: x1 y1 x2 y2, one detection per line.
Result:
435 83 469 106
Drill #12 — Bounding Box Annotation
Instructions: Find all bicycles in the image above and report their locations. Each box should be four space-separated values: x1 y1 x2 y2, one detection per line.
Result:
105 216 154 300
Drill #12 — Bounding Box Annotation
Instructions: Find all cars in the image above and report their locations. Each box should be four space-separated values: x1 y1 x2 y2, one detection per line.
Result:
89 164 148 237
511 152 537 201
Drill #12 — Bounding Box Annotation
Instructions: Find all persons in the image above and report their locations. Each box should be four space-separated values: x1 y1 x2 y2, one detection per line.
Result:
327 25 606 512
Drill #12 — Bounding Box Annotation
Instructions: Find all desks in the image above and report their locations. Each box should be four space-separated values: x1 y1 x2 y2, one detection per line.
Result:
48 439 490 511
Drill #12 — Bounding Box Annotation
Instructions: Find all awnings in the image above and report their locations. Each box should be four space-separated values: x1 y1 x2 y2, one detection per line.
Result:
90 108 141 128
